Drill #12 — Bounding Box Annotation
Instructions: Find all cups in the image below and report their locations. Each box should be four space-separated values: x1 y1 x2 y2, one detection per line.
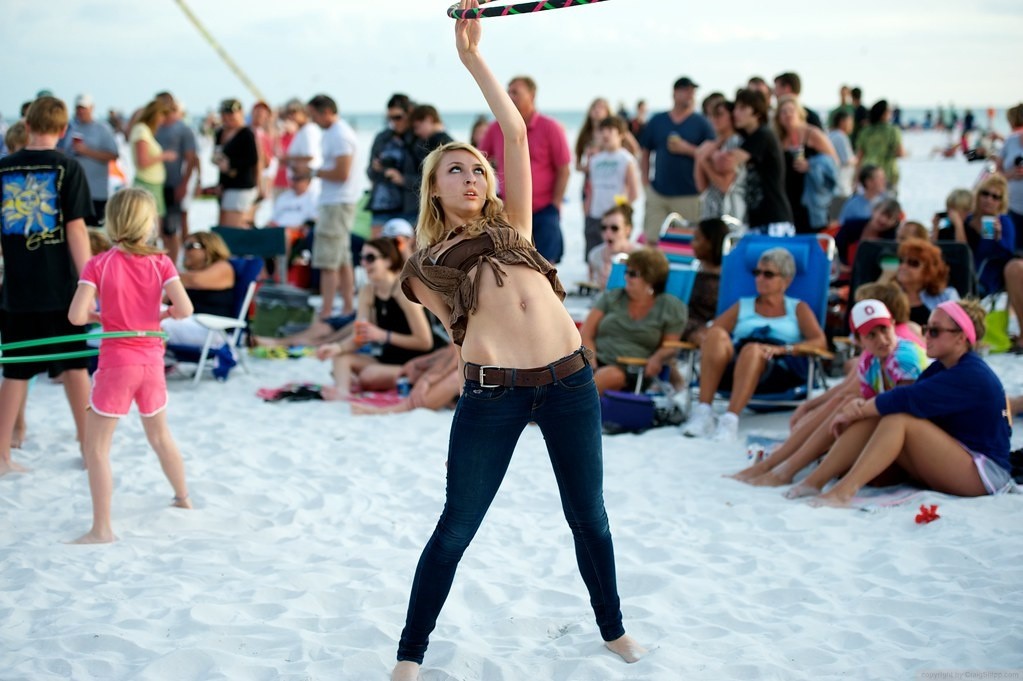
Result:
794 150 805 160
981 213 996 239
71 137 84 151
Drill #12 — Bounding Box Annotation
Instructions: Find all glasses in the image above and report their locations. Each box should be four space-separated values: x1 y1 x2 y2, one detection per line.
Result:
598 223 621 233
752 268 785 280
622 268 644 279
386 109 406 123
183 241 204 252
920 323 961 337
357 252 384 264
979 189 1003 202
896 257 924 268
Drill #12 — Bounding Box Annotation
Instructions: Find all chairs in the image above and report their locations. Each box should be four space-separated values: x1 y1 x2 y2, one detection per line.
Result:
157 245 267 384
578 210 1023 431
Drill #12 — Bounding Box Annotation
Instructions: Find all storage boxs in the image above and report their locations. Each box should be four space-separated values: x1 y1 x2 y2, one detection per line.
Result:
254 283 314 336
597 389 661 425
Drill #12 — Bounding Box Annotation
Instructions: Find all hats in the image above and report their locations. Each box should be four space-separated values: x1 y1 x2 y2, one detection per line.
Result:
219 99 241 114
675 77 699 88
869 99 888 125
848 298 894 337
74 94 93 108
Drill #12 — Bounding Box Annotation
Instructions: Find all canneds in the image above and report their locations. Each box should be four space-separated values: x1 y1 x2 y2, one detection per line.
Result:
396 376 413 400
981 216 998 240
353 320 369 345
669 131 683 141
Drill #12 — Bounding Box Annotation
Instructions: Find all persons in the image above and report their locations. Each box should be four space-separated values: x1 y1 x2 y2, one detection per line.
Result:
0 89 467 474
577 72 1023 507
479 75 571 269
471 120 490 147
68 187 192 543
392 0 646 681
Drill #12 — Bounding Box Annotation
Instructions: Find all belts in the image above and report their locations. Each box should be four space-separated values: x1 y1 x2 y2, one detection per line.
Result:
465 349 595 389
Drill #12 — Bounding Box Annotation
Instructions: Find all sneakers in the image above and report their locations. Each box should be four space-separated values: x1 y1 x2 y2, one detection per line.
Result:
683 404 715 437
712 414 740 442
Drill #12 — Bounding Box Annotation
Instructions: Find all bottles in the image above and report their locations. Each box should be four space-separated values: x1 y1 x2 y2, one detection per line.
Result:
668 131 679 153
396 373 409 399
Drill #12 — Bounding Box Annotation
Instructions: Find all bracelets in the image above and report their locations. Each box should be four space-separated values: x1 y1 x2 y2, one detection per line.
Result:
386 330 391 343
785 344 793 356
311 169 318 176
